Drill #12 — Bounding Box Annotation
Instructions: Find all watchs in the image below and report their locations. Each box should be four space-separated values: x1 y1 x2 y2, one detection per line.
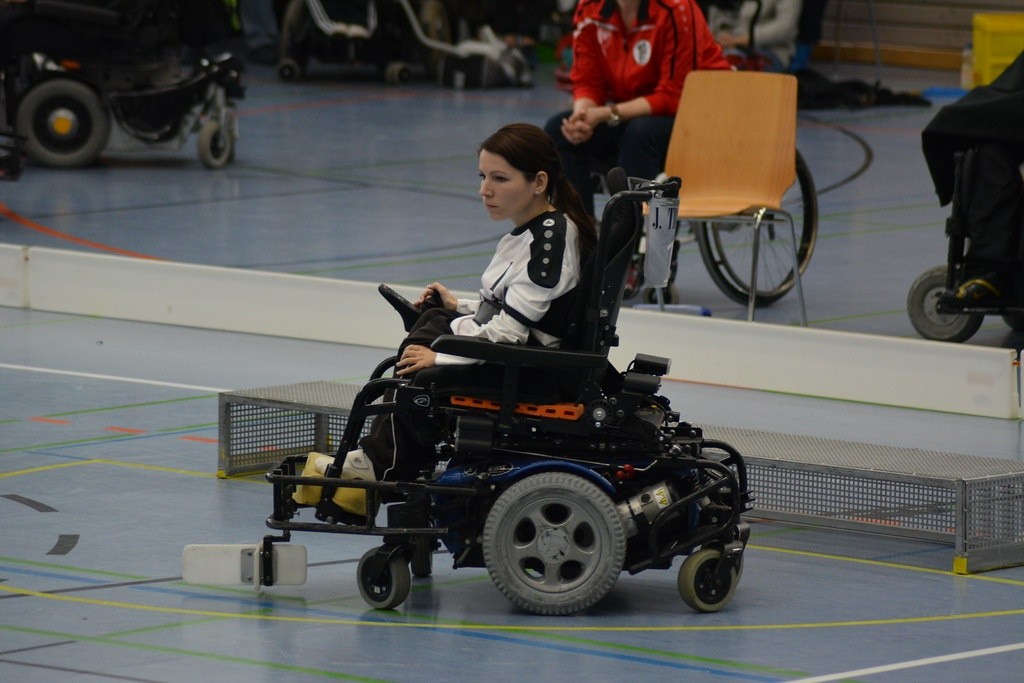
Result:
607 102 620 127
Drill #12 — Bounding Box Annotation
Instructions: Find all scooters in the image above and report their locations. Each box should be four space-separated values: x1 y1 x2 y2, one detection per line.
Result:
0 0 250 169
267 1 546 87
179 152 758 615
906 95 1024 344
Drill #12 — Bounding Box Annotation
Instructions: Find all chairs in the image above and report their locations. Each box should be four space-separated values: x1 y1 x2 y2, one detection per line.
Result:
663 70 808 328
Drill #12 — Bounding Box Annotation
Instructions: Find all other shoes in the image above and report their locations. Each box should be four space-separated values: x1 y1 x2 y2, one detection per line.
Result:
943 269 1010 306
622 256 643 301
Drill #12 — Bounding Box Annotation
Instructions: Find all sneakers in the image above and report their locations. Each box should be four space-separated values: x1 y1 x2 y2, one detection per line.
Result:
315 447 376 482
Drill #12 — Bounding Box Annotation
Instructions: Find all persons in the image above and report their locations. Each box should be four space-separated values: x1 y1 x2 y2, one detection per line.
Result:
302 125 586 495
708 0 800 79
543 0 739 301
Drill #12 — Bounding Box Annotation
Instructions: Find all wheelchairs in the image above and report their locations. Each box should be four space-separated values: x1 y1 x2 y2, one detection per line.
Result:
608 131 820 306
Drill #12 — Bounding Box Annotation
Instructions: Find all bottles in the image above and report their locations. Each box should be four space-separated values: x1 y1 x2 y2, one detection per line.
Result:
962 41 973 84
633 303 712 317
